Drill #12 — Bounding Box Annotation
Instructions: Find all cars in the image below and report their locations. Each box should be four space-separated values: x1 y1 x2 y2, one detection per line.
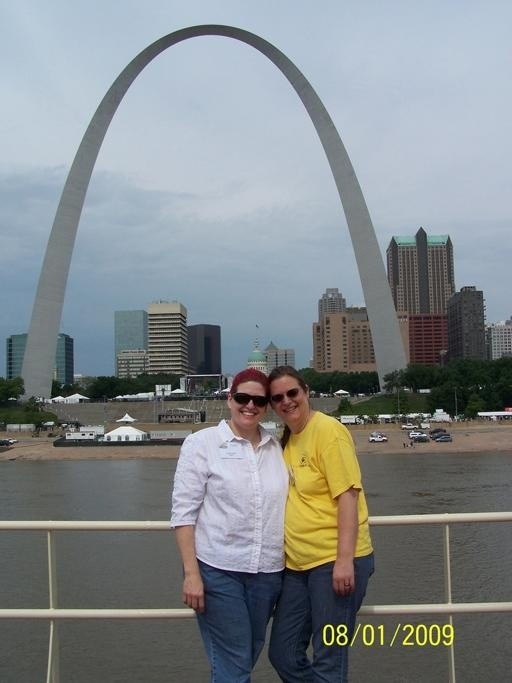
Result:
429 429 453 442
420 420 432 429
0 438 18 446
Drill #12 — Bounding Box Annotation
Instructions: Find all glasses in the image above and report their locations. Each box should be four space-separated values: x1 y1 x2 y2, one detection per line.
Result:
230 392 268 407
270 389 306 402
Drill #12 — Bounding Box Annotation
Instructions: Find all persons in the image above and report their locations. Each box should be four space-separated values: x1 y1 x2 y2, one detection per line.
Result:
401 439 414 448
170 367 290 682
267 365 375 682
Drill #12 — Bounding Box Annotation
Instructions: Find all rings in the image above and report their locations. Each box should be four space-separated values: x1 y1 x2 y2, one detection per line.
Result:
344 583 349 586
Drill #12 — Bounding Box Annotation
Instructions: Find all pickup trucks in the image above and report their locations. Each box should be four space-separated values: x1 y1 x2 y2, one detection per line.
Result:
370 432 388 442
402 424 418 430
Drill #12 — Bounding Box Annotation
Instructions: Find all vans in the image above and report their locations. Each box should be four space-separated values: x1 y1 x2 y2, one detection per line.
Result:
408 432 429 444
